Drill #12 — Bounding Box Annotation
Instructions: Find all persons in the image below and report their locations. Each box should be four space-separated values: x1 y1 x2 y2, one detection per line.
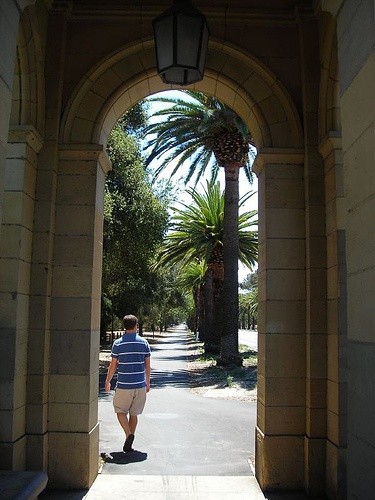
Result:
105 314 151 453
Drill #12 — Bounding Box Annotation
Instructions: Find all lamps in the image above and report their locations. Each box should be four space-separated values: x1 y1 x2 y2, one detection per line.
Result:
152 1 210 86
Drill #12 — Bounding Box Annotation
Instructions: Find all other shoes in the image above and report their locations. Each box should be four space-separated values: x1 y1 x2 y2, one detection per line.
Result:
123 434 134 453
